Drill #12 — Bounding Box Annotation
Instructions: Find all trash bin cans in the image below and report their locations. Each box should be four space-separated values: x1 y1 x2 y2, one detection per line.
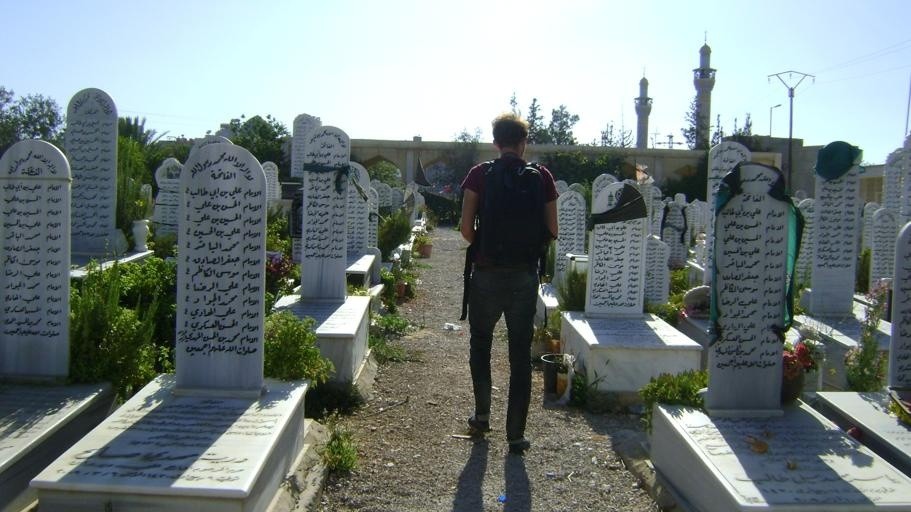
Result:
541 353 576 407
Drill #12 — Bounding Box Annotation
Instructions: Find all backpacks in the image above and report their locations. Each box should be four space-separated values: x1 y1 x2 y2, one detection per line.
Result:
474 159 545 267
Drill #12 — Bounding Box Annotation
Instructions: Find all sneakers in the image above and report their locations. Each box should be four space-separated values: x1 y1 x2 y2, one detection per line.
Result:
469 416 489 428
509 438 530 451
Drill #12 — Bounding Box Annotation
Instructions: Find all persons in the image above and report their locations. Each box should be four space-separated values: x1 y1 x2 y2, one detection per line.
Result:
459 111 561 447
